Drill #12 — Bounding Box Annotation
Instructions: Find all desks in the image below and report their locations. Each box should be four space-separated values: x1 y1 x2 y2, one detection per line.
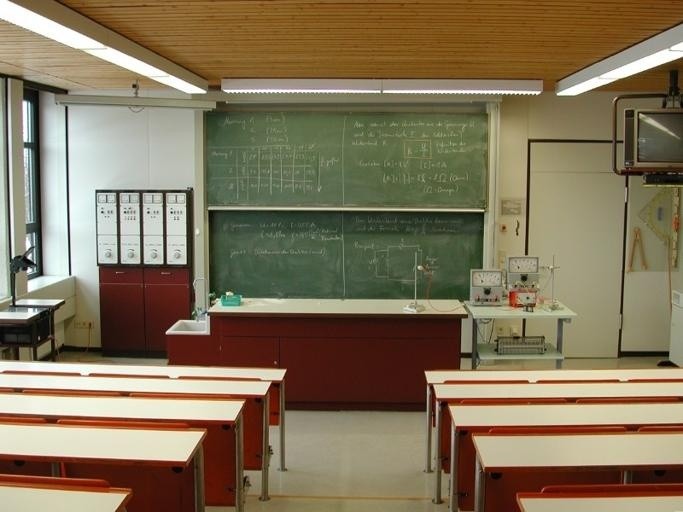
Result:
422 366 682 510
463 298 578 370
1 356 287 510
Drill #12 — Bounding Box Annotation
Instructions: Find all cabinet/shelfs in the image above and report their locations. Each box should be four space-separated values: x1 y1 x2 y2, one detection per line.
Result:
99 268 195 360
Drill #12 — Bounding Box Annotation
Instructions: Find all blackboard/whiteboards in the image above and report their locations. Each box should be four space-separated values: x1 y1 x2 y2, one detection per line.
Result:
195 103 500 298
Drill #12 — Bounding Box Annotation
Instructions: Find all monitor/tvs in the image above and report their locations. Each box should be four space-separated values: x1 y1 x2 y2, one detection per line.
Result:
623 107 683 169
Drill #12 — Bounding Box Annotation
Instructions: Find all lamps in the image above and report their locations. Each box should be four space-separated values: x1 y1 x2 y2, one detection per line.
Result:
553 22 682 99
5 0 218 111
218 76 545 98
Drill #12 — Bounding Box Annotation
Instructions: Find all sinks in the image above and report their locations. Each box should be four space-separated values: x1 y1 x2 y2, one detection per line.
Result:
165 319 211 336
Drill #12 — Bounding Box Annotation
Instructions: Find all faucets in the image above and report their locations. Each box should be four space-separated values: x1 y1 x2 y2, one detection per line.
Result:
192 306 207 322
